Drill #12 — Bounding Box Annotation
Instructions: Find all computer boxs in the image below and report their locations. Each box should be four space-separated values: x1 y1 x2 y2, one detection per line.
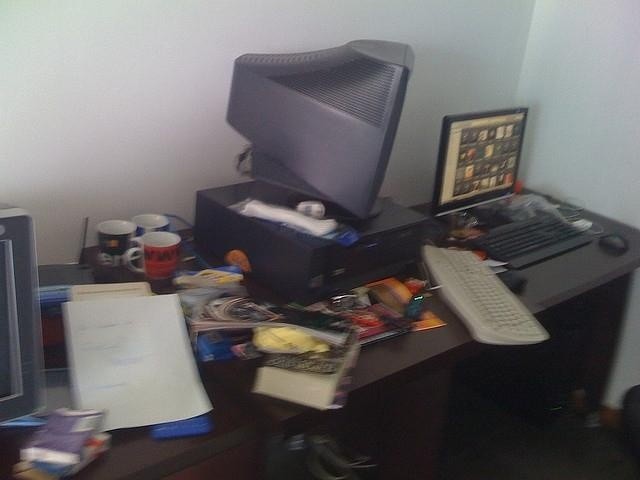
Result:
195 179 428 306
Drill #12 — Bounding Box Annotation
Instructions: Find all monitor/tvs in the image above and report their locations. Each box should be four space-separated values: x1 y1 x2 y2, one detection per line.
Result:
431 106 529 225
226 40 414 216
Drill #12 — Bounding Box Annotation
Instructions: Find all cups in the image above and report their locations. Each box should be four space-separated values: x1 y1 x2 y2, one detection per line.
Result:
96 212 182 281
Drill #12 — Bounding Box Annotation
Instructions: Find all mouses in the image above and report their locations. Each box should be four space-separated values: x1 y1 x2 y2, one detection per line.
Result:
600 235 627 252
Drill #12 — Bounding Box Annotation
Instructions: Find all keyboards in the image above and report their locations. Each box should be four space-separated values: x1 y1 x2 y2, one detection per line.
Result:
420 245 551 345
466 213 592 269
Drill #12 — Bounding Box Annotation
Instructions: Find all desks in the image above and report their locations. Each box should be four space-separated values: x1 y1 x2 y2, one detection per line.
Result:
0 188 640 480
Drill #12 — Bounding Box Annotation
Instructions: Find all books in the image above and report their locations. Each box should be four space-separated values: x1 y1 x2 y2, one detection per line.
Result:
11 264 445 480
